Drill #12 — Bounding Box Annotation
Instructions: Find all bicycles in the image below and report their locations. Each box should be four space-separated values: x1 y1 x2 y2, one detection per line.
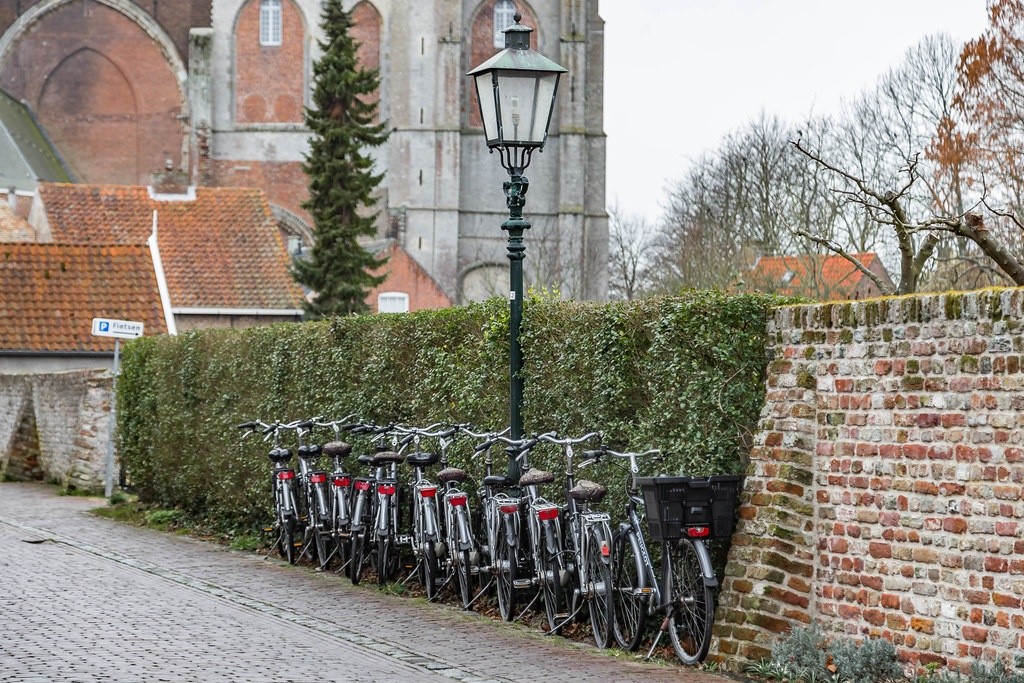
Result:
579 444 720 667
518 431 616 650
261 414 615 651
239 418 309 566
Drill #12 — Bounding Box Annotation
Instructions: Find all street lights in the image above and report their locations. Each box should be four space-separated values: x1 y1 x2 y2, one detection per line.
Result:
465 12 571 615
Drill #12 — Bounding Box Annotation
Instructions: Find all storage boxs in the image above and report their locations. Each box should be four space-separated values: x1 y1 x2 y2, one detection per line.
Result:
637 477 739 540
